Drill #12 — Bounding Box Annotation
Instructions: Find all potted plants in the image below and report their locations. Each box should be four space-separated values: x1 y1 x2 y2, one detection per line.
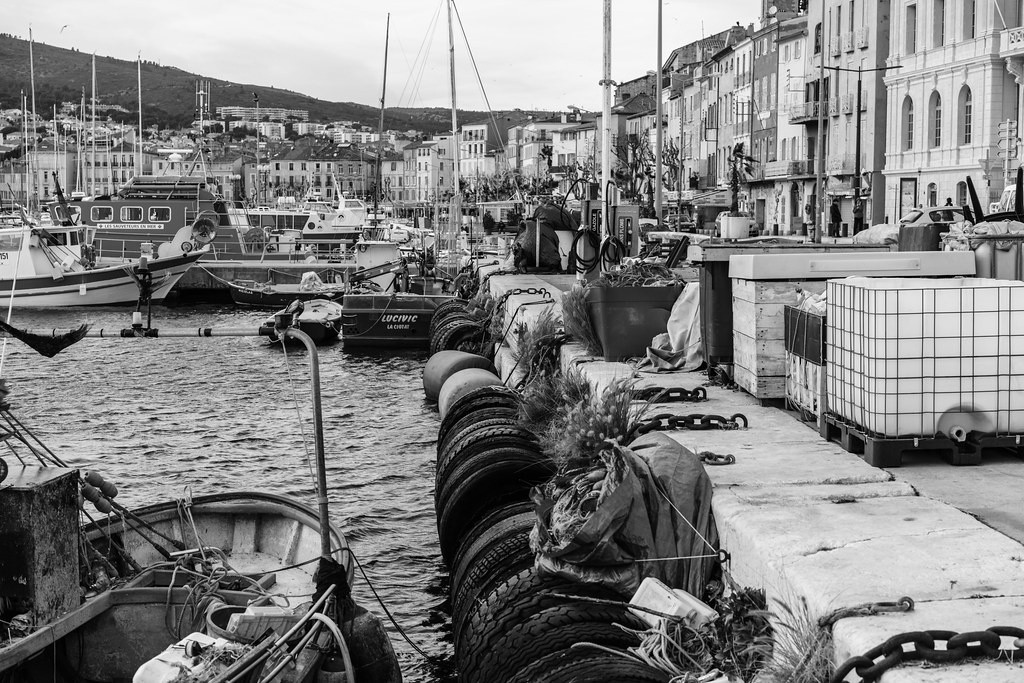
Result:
720 142 760 239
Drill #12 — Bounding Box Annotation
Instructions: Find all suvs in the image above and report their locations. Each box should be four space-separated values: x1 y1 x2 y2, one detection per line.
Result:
898 206 976 229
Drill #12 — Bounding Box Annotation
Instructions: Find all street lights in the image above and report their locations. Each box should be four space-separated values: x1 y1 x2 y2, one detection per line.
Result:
816 65 904 206
646 70 723 232
252 92 260 207
459 148 496 205
425 163 453 187
567 105 625 183
515 126 563 195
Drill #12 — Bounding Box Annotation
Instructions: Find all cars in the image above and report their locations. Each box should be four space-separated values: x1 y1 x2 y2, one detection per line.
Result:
662 215 696 232
715 211 759 238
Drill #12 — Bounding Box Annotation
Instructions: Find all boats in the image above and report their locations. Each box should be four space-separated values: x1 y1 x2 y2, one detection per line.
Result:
0 263 360 683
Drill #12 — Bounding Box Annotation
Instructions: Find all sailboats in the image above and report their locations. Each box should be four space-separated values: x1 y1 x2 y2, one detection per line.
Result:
0 0 683 364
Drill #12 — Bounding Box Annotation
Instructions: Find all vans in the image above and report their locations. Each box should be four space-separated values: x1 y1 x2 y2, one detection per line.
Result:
692 204 730 230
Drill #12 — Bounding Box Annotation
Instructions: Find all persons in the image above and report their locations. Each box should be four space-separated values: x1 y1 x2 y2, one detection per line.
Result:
942 196 954 220
497 221 506 234
482 209 519 236
464 224 469 233
829 198 842 237
853 197 864 235
460 222 464 230
